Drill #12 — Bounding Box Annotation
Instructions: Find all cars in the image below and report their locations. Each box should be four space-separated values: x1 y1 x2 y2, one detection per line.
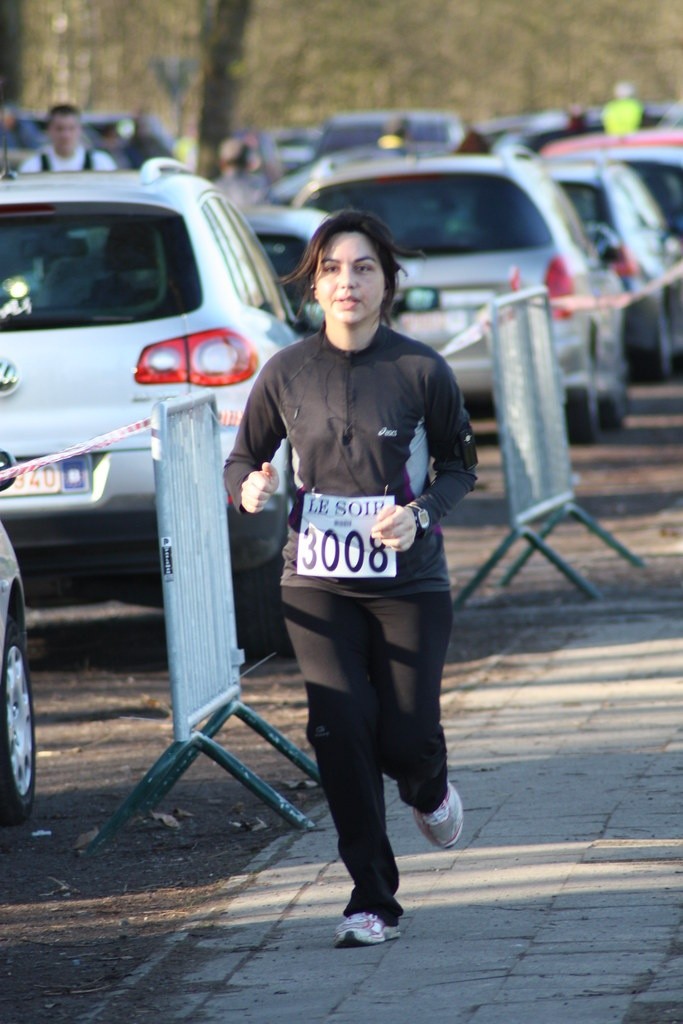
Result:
546 150 683 383
0 453 52 826
0 104 683 249
240 204 349 315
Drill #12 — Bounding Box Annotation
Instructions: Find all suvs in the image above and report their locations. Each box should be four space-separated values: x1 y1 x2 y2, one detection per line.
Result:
283 138 640 446
0 159 312 630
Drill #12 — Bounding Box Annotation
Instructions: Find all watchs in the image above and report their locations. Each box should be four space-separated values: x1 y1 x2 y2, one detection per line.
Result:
406 503 430 540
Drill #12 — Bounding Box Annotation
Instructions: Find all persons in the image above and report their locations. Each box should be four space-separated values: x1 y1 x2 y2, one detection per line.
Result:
213 139 268 193
226 209 480 947
114 105 169 169
18 103 117 249
378 117 410 148
566 104 585 135
601 81 644 135
0 99 43 170
176 140 208 176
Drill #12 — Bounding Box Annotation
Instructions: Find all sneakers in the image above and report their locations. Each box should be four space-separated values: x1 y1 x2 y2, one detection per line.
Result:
333 912 401 947
413 779 464 847
